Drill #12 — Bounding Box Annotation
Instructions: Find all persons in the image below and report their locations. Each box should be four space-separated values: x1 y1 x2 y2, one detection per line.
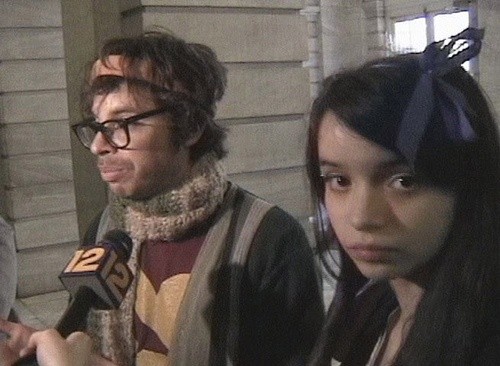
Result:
296 27 500 366
49 24 327 366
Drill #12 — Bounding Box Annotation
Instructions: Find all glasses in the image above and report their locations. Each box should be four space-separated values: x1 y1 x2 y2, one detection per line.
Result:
71 108 166 150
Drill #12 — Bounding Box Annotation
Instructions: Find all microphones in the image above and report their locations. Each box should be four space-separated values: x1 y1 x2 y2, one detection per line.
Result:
12 229 135 366
